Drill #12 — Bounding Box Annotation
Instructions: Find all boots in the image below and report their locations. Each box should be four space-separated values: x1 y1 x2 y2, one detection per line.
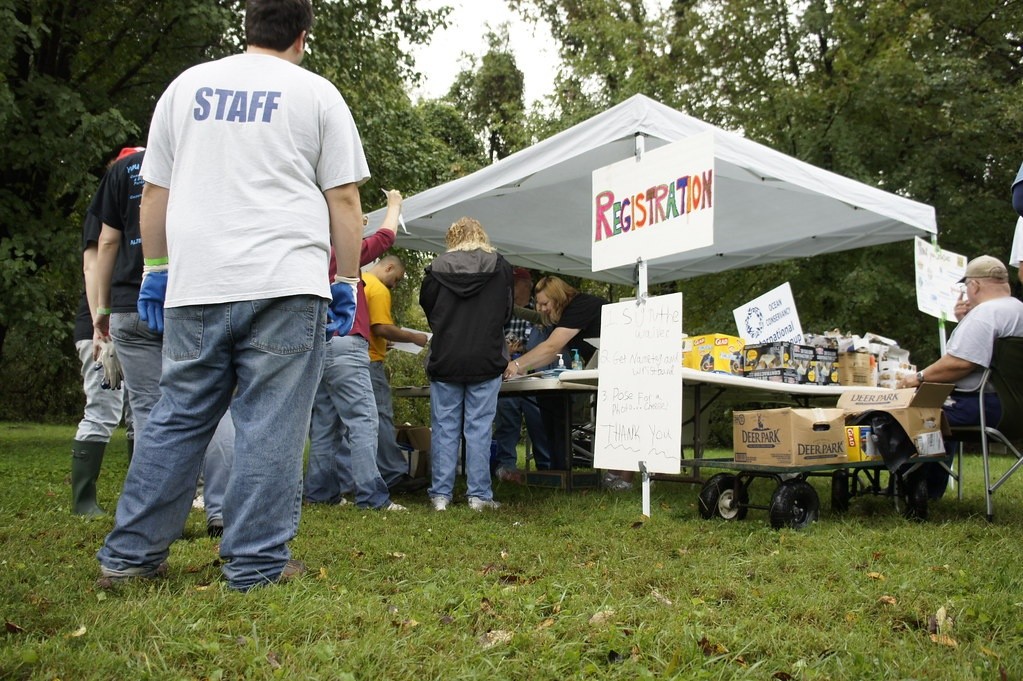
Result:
71 438 107 519
127 438 134 465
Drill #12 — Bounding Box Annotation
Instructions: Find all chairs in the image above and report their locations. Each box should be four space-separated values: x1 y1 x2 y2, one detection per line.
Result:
893 336 1023 522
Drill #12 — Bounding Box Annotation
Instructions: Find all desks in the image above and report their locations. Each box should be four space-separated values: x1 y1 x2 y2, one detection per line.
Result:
394 377 604 495
558 366 919 494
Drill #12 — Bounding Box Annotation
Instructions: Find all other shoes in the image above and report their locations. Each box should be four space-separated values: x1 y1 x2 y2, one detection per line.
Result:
388 502 408 511
339 498 355 507
206 519 224 538
280 559 305 579
388 473 429 495
605 472 632 490
99 562 167 590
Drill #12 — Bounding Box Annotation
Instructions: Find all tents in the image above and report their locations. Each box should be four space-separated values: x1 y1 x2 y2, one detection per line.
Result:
360 92 956 516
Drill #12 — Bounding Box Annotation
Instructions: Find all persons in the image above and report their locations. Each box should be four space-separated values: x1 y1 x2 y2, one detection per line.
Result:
1008 216 1023 282
417 217 519 510
66 135 237 535
875 254 1023 501
304 188 429 512
93 0 373 593
489 264 633 489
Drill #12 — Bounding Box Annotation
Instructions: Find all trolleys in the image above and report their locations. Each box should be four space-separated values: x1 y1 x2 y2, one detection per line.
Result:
679 454 952 533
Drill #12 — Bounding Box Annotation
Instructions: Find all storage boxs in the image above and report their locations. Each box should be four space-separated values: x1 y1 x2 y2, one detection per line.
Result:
397 331 956 491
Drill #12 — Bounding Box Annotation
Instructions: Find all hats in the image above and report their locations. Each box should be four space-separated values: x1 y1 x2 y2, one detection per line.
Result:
956 255 1008 284
512 267 531 281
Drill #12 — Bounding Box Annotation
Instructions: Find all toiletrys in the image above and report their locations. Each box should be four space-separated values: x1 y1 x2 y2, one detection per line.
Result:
557 353 566 369
571 348 582 370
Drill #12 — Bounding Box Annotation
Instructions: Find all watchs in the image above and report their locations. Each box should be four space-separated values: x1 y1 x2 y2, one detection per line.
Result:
915 371 924 383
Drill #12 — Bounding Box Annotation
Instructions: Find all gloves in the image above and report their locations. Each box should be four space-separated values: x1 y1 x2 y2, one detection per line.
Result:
93 335 124 391
325 274 360 342
137 256 170 333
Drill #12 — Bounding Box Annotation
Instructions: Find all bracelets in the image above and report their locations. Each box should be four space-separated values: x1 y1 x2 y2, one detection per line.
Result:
96 307 110 315
513 360 519 367
143 255 168 266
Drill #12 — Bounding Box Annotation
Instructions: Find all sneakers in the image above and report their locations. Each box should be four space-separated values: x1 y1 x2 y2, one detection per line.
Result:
468 496 501 511
430 496 449 511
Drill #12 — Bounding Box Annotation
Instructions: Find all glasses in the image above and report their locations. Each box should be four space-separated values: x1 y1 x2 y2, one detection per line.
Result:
960 279 980 293
